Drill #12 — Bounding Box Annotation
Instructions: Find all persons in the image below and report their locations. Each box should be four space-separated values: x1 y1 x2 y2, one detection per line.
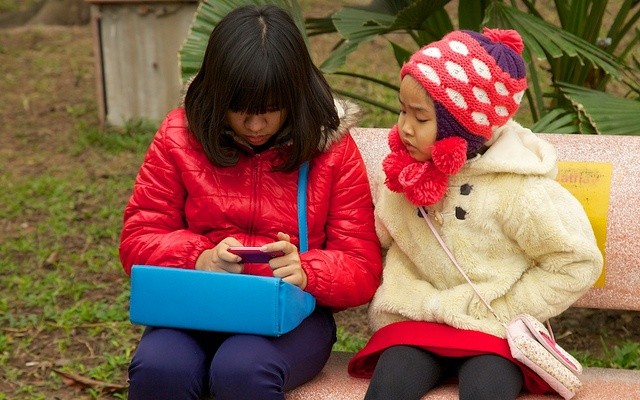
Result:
348 26 604 400
118 5 383 400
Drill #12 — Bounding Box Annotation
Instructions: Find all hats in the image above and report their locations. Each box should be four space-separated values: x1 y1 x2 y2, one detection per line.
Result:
383 28 529 207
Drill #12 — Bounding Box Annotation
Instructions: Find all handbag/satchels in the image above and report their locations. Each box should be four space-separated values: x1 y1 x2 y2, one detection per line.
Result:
130 263 315 338
505 315 584 399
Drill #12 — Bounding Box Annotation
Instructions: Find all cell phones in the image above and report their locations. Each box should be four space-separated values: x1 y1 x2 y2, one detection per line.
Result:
228 247 285 264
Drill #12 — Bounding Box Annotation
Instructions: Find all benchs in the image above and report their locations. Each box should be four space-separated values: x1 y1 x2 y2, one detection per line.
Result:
285 126 639 399
